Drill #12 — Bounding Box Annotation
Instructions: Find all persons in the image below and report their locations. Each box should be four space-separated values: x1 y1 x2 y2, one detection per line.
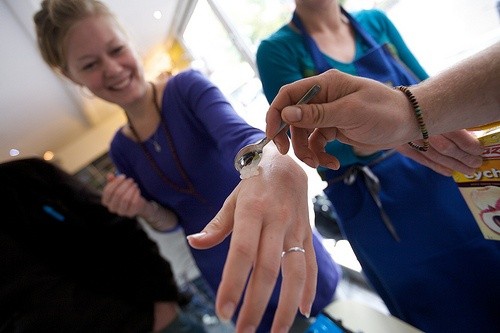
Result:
268 38 500 170
34 1 343 333
0 158 207 333
257 1 500 333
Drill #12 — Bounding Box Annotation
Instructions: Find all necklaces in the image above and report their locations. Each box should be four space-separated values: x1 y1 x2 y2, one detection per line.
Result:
124 82 214 215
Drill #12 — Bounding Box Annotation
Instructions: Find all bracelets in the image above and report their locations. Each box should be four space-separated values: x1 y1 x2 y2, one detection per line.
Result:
393 85 430 151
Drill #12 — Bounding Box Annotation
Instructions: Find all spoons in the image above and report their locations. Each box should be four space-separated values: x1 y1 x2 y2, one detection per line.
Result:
234 84 320 172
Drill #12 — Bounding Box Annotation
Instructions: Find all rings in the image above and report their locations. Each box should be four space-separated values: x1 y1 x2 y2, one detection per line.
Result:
281 246 305 258
